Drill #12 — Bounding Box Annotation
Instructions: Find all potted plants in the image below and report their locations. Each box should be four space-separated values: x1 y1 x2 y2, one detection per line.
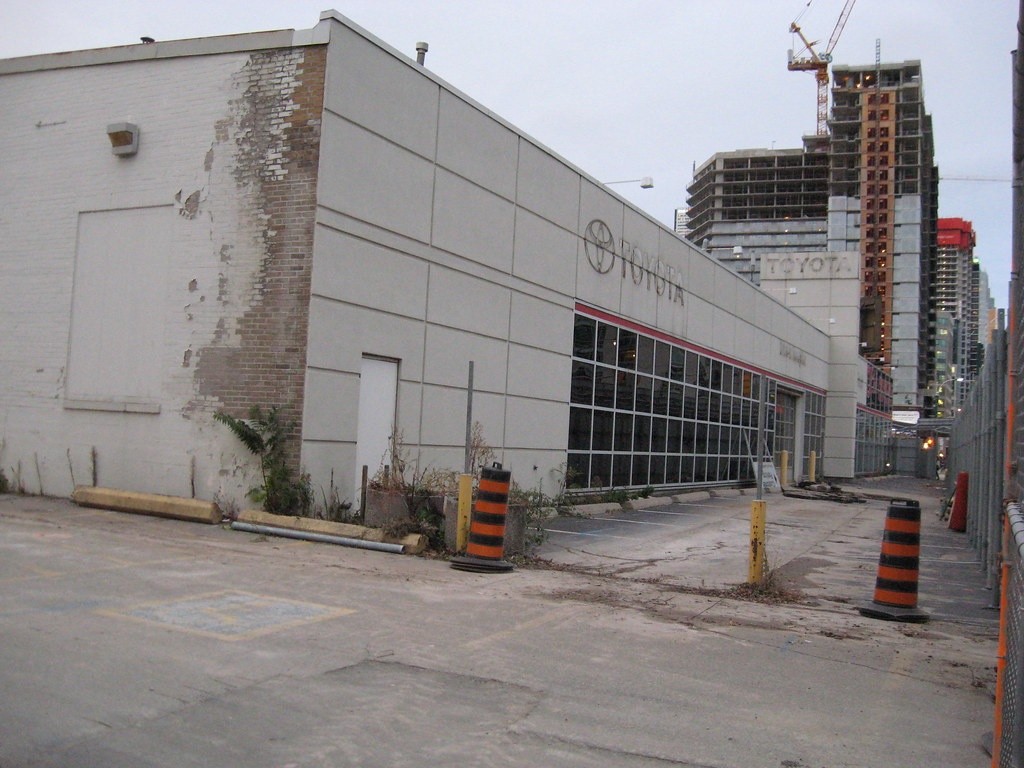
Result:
365 428 450 532
444 424 532 557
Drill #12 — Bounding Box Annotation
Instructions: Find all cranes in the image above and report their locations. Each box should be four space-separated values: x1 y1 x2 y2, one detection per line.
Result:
786 0 857 138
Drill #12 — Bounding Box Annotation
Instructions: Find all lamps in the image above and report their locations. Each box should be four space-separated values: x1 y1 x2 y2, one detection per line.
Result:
867 357 885 362
808 319 836 324
604 177 654 191
859 342 867 347
705 246 743 260
105 122 137 156
881 367 896 370
764 287 797 294
